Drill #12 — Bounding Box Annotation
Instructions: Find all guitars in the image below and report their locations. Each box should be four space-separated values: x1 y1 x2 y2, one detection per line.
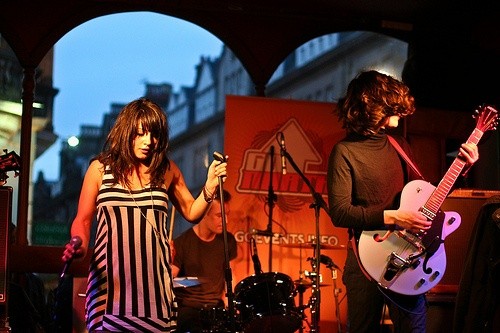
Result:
0 149 24 184
358 105 500 296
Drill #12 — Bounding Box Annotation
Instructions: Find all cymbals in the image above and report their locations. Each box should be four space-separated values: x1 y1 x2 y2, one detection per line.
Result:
277 241 348 250
172 279 206 288
293 280 331 287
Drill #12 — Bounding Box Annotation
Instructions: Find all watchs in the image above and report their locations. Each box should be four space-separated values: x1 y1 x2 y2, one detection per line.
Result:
203 186 213 203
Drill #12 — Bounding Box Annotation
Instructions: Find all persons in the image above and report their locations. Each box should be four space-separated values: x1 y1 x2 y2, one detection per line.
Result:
62 97 229 333
327 70 480 332
172 188 238 333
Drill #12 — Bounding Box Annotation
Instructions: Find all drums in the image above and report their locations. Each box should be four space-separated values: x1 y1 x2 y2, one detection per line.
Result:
234 273 302 333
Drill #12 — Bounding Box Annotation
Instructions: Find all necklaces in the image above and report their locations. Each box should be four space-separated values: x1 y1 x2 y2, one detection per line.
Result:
115 163 163 236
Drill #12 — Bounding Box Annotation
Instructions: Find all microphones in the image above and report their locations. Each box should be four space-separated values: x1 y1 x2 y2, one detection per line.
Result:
252 238 261 272
250 228 283 237
319 255 339 269
60 236 82 279
281 133 287 174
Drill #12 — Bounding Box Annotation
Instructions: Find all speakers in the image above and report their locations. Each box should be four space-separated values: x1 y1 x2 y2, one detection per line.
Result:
423 190 500 333
0 185 13 302
